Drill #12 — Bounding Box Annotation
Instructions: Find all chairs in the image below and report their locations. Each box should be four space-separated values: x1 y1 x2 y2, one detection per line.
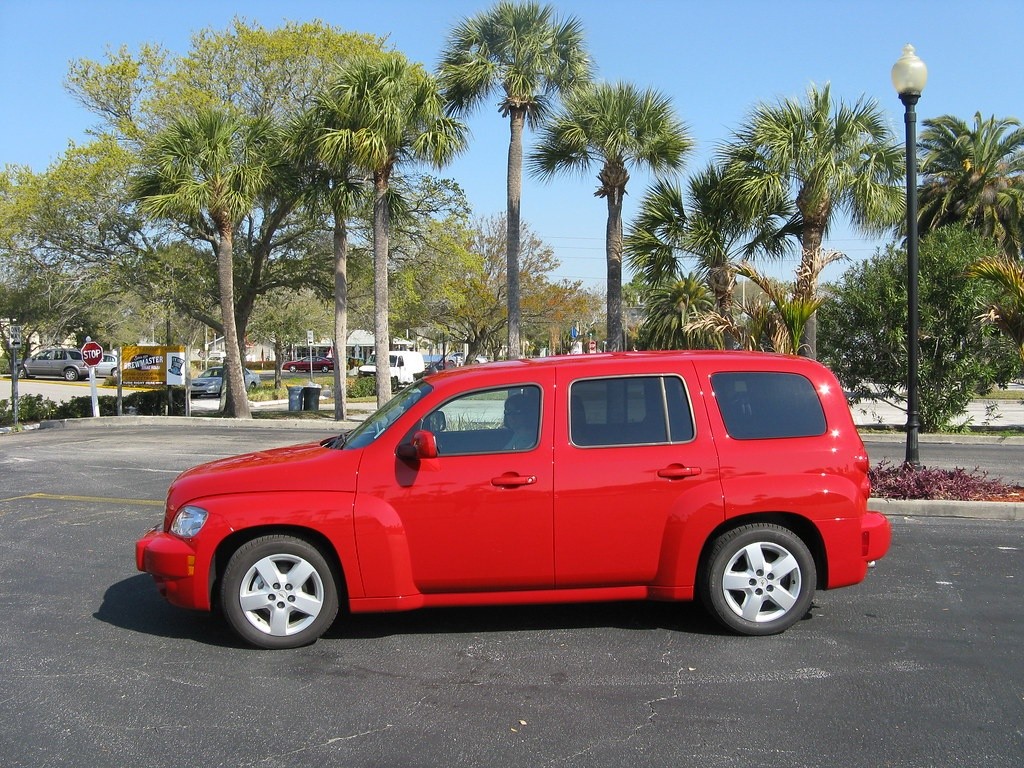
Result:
738 371 793 437
572 395 589 445
642 380 690 442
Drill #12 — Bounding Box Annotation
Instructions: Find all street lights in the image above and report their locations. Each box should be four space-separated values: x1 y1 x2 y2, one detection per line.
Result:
890 42 928 472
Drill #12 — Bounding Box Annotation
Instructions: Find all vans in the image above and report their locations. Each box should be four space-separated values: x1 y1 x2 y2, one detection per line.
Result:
358 350 426 392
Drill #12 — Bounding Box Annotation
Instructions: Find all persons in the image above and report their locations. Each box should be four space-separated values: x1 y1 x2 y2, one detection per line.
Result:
501 393 535 449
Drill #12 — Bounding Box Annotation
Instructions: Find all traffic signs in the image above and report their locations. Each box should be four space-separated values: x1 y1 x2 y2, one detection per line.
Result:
9 326 21 348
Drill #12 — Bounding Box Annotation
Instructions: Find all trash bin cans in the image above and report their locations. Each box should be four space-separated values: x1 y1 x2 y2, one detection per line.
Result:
304 385 321 412
287 385 305 413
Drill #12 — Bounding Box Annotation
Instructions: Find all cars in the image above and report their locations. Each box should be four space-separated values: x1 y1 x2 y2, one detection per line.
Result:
15 347 119 381
448 352 488 364
136 348 898 650
191 367 262 399
282 356 334 373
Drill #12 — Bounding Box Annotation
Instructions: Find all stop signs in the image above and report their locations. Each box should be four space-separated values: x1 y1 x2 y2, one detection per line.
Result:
81 342 104 366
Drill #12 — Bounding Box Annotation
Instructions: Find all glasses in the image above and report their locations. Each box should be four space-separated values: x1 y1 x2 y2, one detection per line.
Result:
504 408 526 416
